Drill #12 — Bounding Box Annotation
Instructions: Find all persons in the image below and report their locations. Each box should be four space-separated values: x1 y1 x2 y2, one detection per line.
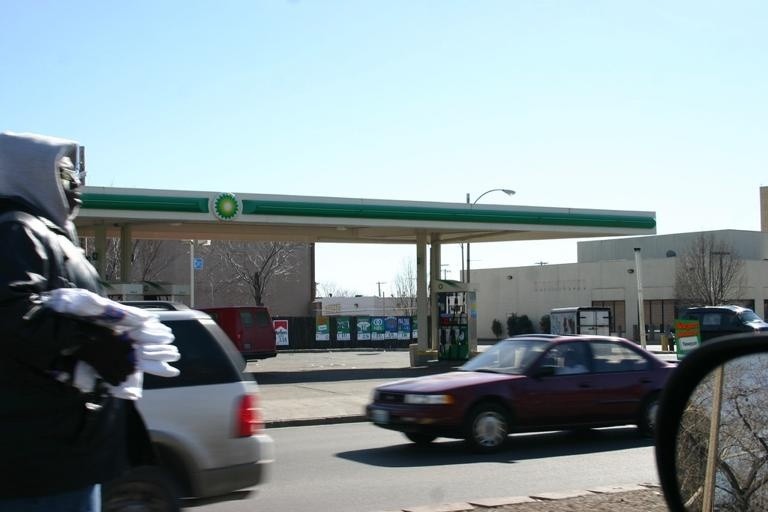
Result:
667 329 676 352
1 130 147 512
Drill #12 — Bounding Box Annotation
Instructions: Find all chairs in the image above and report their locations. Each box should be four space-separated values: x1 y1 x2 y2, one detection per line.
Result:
592 356 647 372
527 350 547 365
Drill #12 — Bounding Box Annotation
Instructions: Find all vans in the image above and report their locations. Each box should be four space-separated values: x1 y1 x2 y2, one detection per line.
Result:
197 306 277 373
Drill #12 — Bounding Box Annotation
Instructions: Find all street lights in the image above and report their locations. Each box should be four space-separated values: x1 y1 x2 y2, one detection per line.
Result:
460 188 515 285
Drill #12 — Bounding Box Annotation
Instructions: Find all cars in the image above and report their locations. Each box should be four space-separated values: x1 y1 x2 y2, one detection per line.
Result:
680 305 767 344
100 300 273 510
367 333 680 456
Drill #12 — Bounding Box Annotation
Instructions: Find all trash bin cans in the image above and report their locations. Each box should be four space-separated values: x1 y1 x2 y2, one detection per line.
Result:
661 333 668 351
409 344 417 367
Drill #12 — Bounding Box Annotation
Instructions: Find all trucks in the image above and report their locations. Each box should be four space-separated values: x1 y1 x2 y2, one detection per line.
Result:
543 306 611 354
